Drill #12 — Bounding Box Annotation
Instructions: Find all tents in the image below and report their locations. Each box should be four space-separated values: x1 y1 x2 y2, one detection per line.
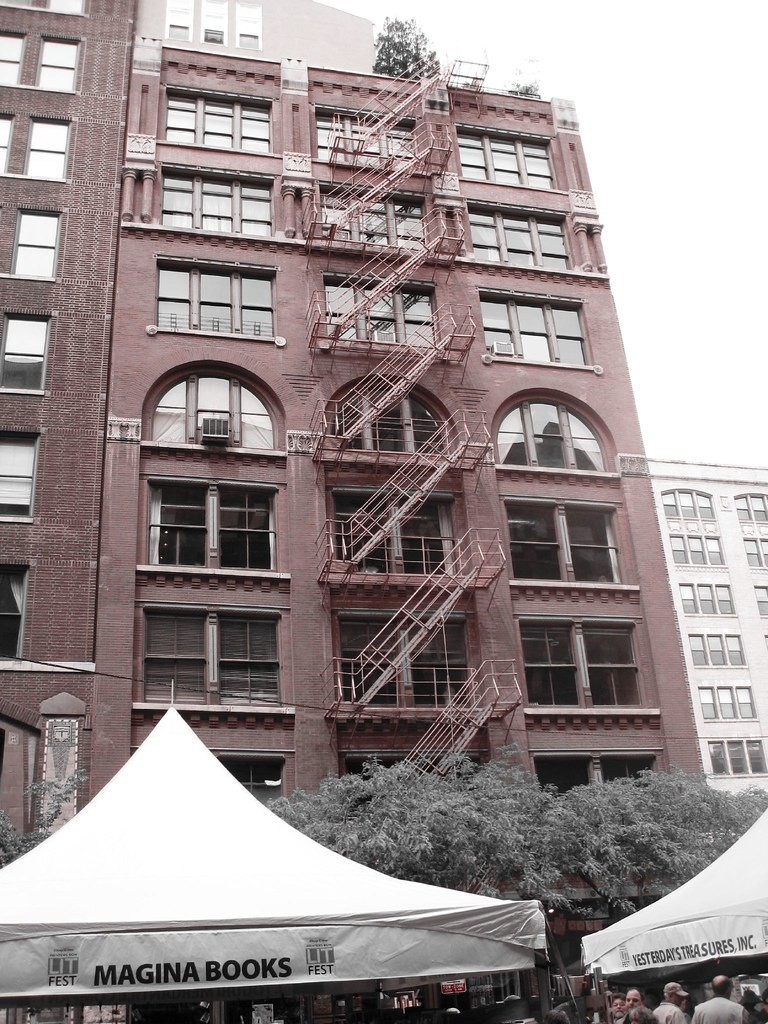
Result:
580 806 768 976
0 707 552 1021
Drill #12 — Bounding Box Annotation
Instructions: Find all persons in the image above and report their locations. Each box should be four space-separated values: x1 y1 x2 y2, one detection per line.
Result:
612 974 768 1024
543 1010 570 1024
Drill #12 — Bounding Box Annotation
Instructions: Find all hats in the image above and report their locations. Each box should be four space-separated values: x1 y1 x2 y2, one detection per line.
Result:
738 987 764 1003
663 982 689 996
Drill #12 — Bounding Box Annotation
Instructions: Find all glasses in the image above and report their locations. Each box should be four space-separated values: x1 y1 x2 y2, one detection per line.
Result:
626 998 641 1002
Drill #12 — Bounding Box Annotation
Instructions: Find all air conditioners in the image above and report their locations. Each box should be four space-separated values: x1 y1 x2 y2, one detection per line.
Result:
199 418 230 441
489 342 515 356
